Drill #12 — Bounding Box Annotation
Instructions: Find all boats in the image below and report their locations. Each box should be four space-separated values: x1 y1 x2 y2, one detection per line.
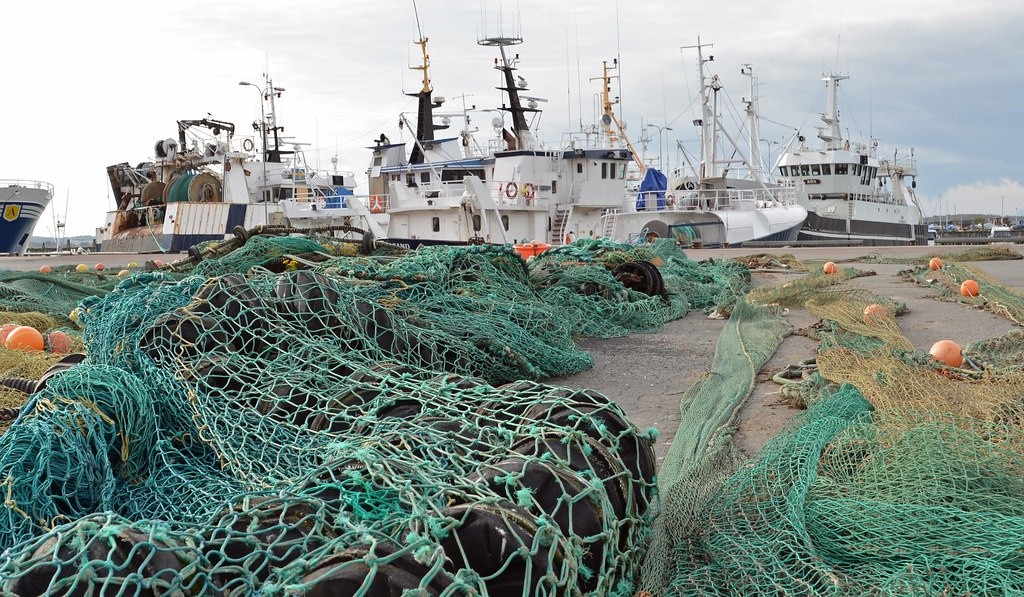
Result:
87 0 935 253
0 175 56 258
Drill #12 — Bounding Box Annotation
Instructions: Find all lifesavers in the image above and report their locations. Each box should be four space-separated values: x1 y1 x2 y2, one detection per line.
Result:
370 194 383 212
243 139 253 151
666 194 675 206
505 182 517 199
522 183 534 199
